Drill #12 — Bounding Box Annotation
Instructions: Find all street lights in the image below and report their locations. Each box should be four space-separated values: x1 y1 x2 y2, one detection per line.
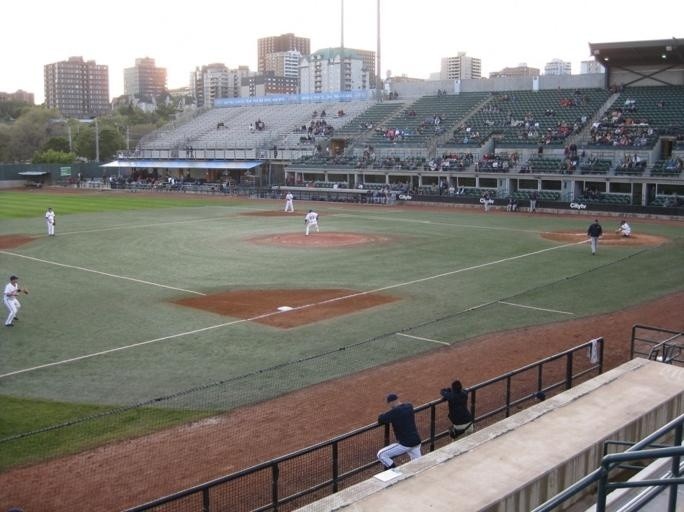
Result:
450 426 465 438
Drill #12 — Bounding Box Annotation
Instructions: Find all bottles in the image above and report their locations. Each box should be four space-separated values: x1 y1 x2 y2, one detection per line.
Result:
10 275 18 280
532 392 546 400
387 394 398 402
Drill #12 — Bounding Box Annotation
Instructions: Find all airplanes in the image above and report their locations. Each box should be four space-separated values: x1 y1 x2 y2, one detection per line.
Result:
53 223 55 225
23 289 28 294
615 229 621 234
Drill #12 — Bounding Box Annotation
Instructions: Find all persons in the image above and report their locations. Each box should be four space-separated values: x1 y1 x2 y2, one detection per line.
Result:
374 393 422 474
303 209 321 235
438 377 473 443
45 205 56 238
107 89 683 215
613 219 633 240
587 216 604 257
3 275 28 328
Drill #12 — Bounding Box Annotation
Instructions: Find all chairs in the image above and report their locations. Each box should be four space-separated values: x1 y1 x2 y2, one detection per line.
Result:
6 317 18 327
134 85 684 208
